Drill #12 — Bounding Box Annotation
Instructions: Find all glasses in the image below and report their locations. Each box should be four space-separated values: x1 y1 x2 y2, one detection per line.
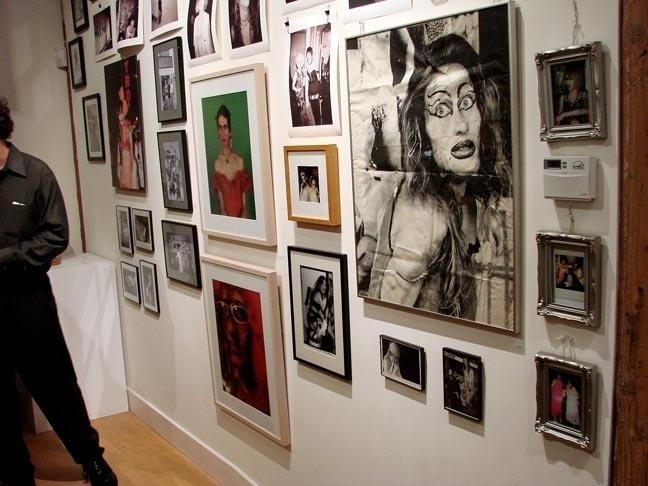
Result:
215 300 249 324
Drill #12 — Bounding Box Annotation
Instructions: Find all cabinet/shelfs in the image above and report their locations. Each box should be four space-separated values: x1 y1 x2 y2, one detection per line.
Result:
29 253 130 435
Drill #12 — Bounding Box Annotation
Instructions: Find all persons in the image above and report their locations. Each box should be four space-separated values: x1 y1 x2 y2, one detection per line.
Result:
445 369 461 407
550 373 565 423
305 276 334 353
229 0 263 49
463 357 476 407
357 33 508 320
565 378 581 425
299 171 309 200
350 27 415 171
291 48 332 125
553 69 589 125
0 94 118 486
211 104 253 218
192 0 216 58
556 255 583 291
384 341 403 379
213 281 266 412
309 175 319 203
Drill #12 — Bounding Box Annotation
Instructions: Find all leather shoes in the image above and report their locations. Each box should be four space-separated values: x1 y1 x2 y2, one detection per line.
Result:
82 458 117 486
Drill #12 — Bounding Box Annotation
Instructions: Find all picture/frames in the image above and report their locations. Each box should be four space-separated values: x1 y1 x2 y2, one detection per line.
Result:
189 63 277 247
116 205 134 254
534 352 598 453
71 0 89 33
442 347 482 422
284 145 341 226
200 253 290 445
380 335 425 391
120 261 140 304
132 208 154 251
288 246 350 380
536 230 602 327
535 41 606 141
343 0 521 336
153 37 186 122
162 220 201 288
139 259 159 313
82 93 105 161
68 37 86 89
157 130 192 210
